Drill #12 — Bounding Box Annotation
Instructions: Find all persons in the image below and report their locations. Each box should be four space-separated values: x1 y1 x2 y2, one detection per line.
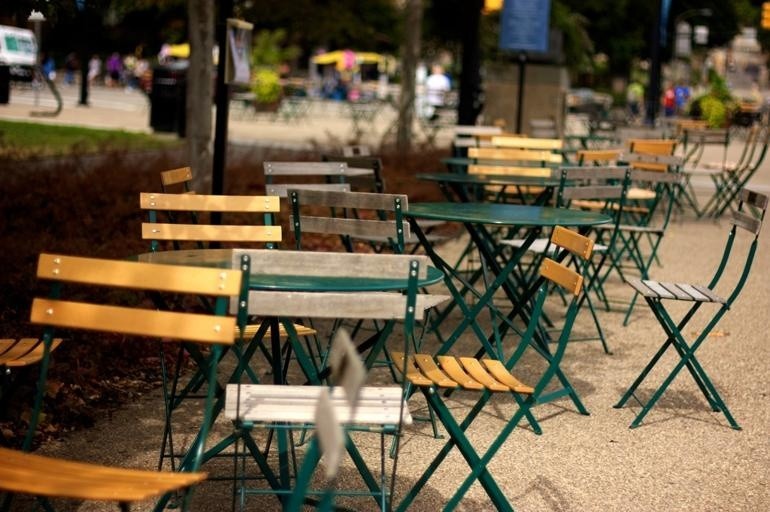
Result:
424 60 454 108
625 74 697 123
41 43 148 96
